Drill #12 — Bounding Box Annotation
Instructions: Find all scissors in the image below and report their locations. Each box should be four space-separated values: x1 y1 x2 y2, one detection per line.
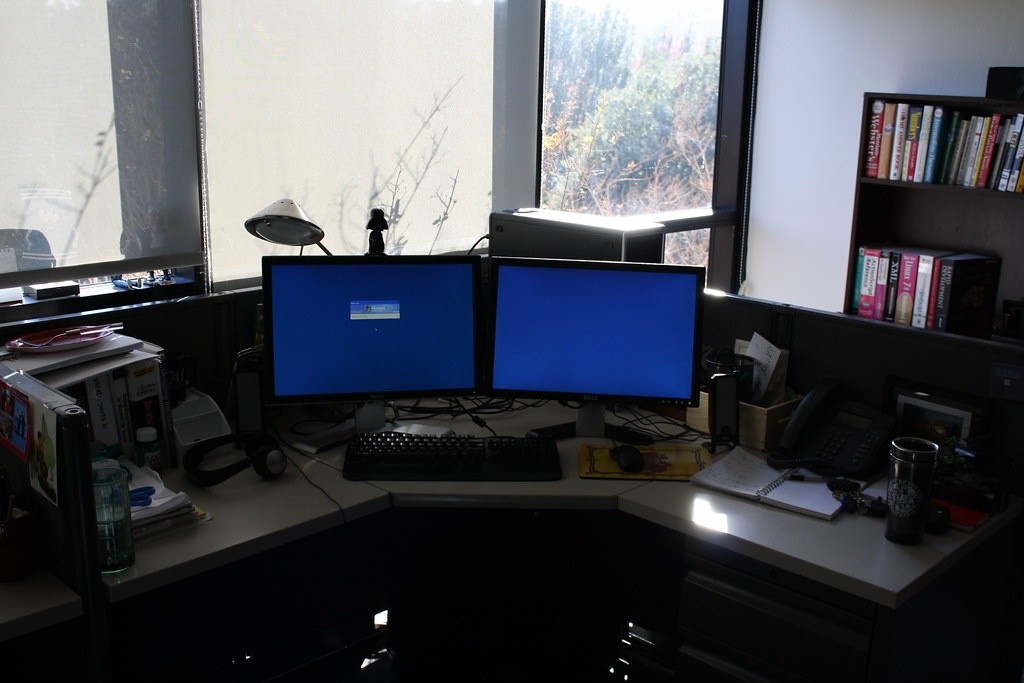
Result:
96 485 155 506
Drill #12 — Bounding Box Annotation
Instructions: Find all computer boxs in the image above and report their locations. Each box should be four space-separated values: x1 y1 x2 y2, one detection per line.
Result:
489 208 666 264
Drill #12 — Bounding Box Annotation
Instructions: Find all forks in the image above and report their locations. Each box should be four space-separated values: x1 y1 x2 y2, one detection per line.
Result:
19 321 124 346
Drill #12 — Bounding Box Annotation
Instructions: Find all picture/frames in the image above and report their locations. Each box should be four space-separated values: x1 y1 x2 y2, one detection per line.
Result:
890 386 975 441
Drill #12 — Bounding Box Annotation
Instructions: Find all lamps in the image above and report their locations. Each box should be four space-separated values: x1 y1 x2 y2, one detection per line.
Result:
245 198 331 257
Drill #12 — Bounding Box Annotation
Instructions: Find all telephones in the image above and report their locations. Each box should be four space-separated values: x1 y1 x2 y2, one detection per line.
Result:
777 379 897 481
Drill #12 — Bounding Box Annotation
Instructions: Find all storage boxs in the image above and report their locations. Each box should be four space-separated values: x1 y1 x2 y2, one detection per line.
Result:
685 386 807 450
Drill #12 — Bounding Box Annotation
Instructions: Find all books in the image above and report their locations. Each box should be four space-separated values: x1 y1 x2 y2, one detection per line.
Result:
87 456 215 545
947 113 1024 194
690 446 841 522
864 98 959 184
60 360 168 463
852 244 1003 338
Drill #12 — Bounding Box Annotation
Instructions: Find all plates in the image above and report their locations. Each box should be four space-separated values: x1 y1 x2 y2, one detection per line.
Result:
6 325 114 353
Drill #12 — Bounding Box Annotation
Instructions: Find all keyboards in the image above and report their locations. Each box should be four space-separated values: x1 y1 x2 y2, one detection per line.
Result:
343 433 563 482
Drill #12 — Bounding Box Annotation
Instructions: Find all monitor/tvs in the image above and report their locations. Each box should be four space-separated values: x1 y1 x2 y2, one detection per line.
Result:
485 255 706 445
261 254 484 455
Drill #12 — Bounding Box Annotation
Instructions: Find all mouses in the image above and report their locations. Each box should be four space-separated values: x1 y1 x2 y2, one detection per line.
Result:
609 445 645 474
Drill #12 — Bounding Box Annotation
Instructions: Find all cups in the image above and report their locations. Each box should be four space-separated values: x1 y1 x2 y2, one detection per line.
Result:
92 466 136 574
885 437 939 545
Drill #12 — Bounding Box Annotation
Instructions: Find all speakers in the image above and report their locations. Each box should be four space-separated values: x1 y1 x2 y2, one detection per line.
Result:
233 361 266 450
701 373 740 454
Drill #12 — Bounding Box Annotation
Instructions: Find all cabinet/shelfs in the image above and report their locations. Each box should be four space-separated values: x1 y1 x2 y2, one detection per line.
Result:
843 92 1024 343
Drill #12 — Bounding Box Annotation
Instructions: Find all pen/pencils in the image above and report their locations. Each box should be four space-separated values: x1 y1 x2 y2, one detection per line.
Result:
785 473 845 482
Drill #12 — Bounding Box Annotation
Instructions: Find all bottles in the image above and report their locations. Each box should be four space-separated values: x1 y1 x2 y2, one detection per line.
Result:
135 425 164 470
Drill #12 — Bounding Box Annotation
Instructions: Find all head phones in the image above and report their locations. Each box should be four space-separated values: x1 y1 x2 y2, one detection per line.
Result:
182 432 287 489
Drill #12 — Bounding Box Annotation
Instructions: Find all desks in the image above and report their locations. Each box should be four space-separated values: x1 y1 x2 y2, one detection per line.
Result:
0 250 1024 683
